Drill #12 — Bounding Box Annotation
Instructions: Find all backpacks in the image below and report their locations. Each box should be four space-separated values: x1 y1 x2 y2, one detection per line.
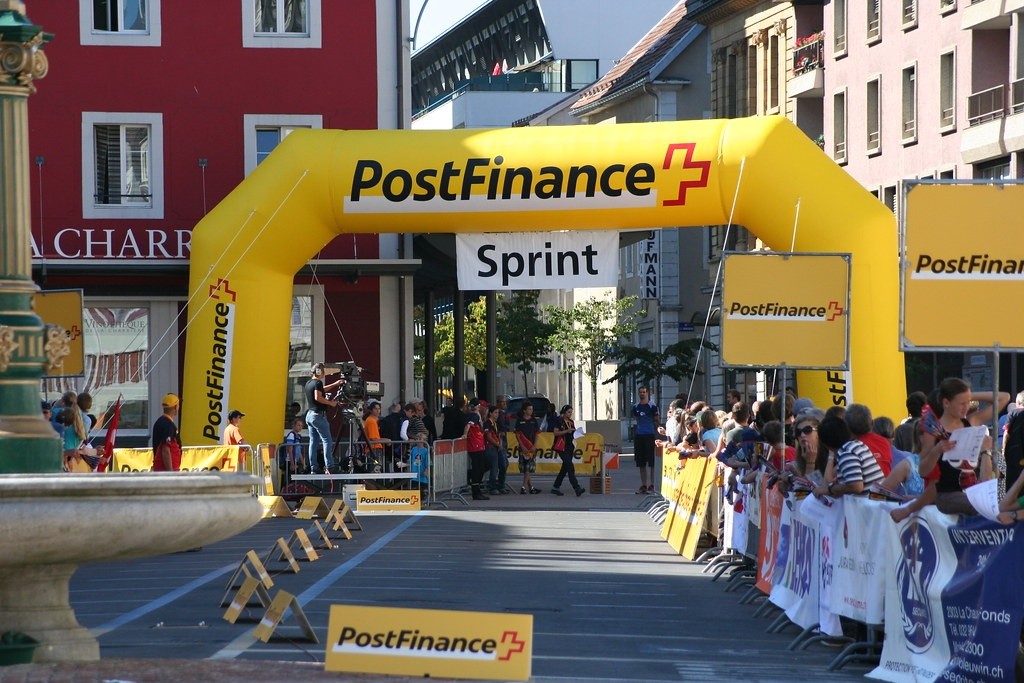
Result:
278 431 302 470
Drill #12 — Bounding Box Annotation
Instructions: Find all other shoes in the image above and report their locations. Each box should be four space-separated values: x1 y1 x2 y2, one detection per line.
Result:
821 636 849 648
311 467 322 475
529 488 541 494
646 486 655 493
635 487 647 494
576 488 585 497
489 489 501 495
474 494 490 501
324 466 344 475
551 489 564 496
521 487 526 494
500 488 509 494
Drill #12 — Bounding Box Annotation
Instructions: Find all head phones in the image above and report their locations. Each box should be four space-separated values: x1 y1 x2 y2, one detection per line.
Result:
315 362 322 376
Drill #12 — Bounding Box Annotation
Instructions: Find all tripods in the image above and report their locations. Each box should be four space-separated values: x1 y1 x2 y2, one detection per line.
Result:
331 408 379 466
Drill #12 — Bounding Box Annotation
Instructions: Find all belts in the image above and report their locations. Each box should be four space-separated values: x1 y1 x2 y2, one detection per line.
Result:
309 410 326 414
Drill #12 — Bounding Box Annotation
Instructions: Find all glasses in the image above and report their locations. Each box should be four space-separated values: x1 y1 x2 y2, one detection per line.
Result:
795 425 817 436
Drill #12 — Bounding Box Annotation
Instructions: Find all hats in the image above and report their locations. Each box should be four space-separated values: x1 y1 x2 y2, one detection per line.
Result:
470 397 480 405
412 398 423 404
161 393 183 408
310 363 325 372
228 410 245 420
1008 391 1024 410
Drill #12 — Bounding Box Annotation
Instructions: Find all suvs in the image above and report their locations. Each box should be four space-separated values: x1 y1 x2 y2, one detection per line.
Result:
497 391 560 434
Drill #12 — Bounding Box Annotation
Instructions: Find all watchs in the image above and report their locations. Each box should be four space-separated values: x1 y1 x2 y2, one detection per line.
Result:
828 482 835 494
1010 511 1017 522
828 456 835 460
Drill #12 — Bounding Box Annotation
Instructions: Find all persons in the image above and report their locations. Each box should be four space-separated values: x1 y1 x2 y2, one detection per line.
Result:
365 401 391 472
305 363 345 475
347 395 509 500
41 391 106 472
539 403 586 496
513 401 541 494
655 378 1024 647
280 419 308 493
224 410 248 445
150 394 182 471
630 386 659 494
